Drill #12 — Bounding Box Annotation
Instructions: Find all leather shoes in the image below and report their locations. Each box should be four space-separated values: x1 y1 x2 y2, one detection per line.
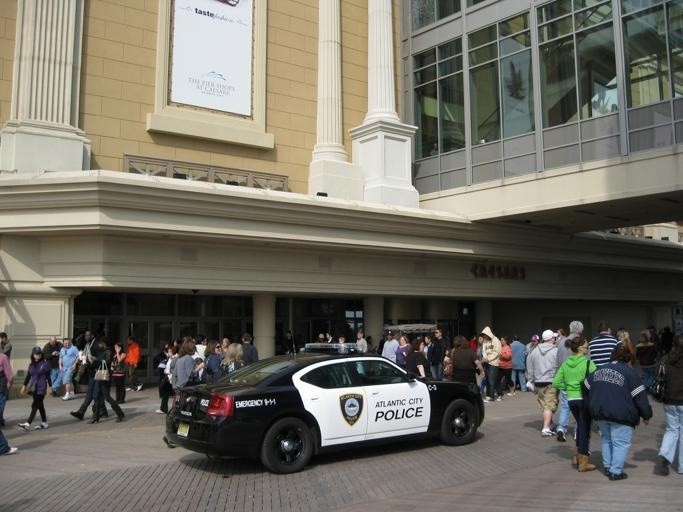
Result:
101 412 108 418
658 455 669 476
70 412 83 420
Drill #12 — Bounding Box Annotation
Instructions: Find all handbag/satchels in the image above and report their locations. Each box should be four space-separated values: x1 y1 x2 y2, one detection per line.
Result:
580 358 591 409
200 356 216 382
95 360 110 382
26 361 47 394
649 357 670 400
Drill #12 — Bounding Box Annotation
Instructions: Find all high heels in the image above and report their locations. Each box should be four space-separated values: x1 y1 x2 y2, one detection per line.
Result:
87 415 102 424
115 413 124 422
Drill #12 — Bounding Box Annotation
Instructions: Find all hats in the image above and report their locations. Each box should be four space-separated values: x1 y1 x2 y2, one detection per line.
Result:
32 347 42 354
319 334 324 339
385 329 394 336
530 334 540 341
542 329 559 340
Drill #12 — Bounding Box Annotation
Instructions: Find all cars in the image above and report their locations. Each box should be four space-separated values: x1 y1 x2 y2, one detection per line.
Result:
164 354 484 473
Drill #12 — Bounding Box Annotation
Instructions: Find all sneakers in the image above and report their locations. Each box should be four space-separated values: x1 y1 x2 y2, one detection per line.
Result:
610 472 628 480
541 429 556 437
156 409 164 414
34 423 49 429
17 422 30 431
5 448 19 455
604 468 610 474
556 429 567 442
62 394 73 400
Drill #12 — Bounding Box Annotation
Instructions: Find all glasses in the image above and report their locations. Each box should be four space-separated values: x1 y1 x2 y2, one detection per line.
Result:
581 345 589 349
215 345 220 349
435 331 440 335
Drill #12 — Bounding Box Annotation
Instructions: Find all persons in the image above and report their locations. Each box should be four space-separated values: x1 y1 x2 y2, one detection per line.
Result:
241 333 258 365
587 322 623 437
480 326 505 402
661 326 674 355
220 342 247 374
582 348 654 481
510 334 528 392
191 357 207 383
110 341 127 404
395 333 412 366
555 320 588 442
41 336 64 396
355 328 368 355
124 336 143 392
452 334 485 388
645 325 660 356
154 345 178 416
555 328 566 347
552 335 599 472
406 338 429 379
59 338 80 401
477 335 494 395
0 351 18 456
381 328 400 364
525 329 561 437
87 336 126 424
432 328 451 377
526 334 541 354
416 334 428 358
172 340 198 408
499 336 517 397
220 338 229 350
0 331 12 359
657 334 683 478
615 328 634 355
280 330 295 356
423 336 436 377
154 343 170 398
316 333 326 343
173 337 182 355
469 336 478 353
442 348 456 377
326 332 335 343
193 333 210 361
201 339 223 383
337 335 350 355
366 336 377 354
429 142 440 156
69 329 109 421
377 332 387 355
635 329 657 392
18 346 53 431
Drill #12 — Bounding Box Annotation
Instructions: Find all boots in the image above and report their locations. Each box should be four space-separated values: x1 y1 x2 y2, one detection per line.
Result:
578 455 596 471
